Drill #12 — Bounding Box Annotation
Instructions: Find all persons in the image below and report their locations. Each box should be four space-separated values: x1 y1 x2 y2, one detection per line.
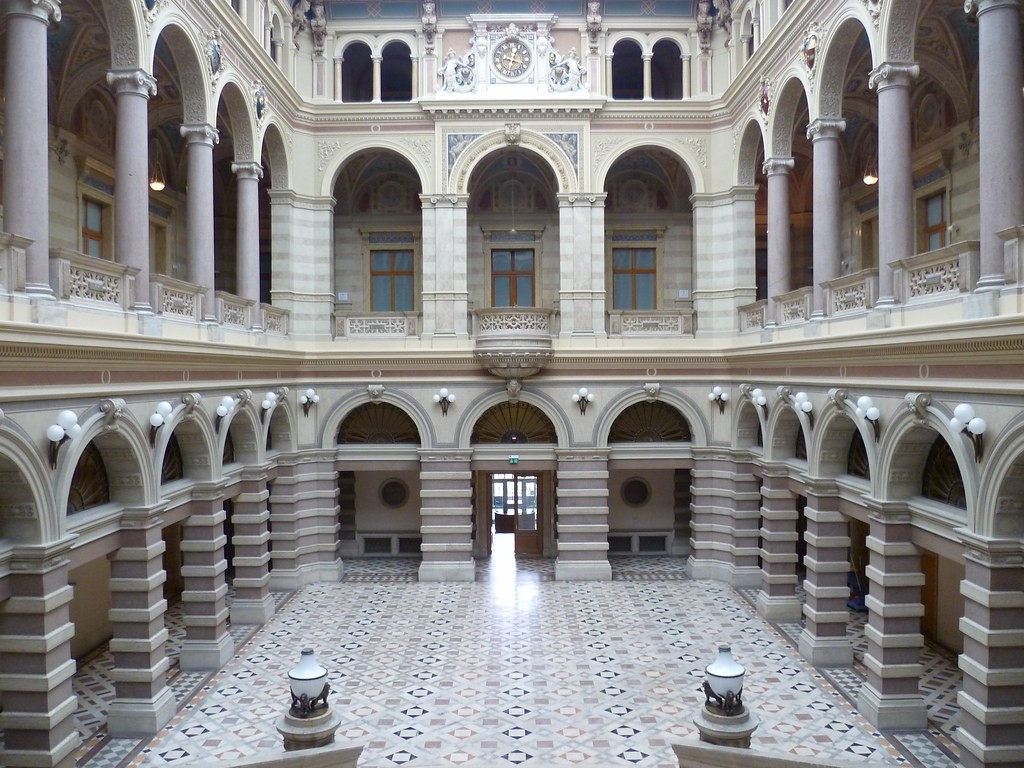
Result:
588 2 601 23
697 3 712 28
422 3 435 24
713 0 724 20
440 48 469 82
294 0 310 25
554 48 583 80
311 5 326 30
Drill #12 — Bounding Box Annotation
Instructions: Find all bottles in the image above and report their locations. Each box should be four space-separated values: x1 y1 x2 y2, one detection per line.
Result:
287 648 328 700
705 644 746 699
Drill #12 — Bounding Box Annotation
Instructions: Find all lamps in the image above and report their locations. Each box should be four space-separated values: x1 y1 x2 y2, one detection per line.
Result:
856 395 880 443
751 389 769 421
46 409 83 471
149 400 173 449
705 645 745 715
794 391 815 431
508 158 517 233
949 404 986 464
287 648 330 716
149 77 166 192
299 388 319 418
862 98 878 186
215 395 236 435
708 386 729 415
432 387 457 417
573 388 594 416
260 392 276 426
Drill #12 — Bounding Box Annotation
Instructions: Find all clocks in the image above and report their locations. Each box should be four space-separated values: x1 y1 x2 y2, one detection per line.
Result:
488 33 537 81
